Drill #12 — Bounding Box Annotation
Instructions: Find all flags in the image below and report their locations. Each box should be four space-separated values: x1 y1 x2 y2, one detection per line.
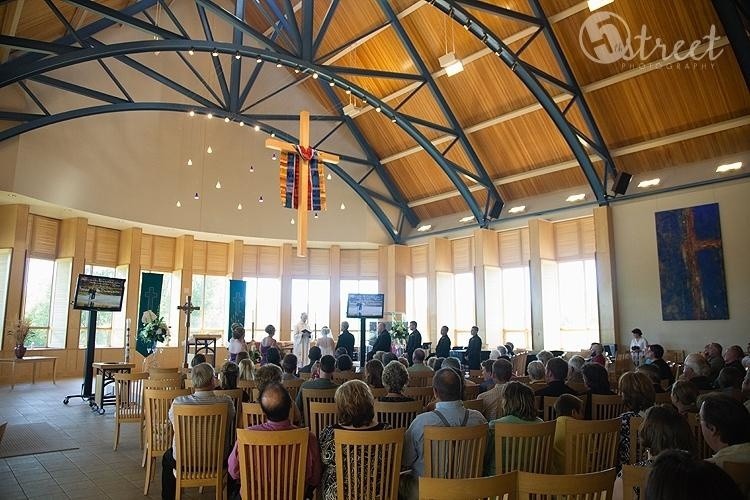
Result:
279 144 326 213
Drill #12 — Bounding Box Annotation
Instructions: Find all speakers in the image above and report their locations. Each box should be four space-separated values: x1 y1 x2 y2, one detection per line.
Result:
488 198 504 219
611 171 634 194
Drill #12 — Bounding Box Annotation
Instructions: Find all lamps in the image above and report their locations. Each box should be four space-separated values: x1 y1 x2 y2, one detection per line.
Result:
342 44 362 122
439 12 466 83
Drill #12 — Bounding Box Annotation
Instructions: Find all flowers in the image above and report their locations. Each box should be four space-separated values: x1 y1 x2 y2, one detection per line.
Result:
387 310 412 341
136 311 171 354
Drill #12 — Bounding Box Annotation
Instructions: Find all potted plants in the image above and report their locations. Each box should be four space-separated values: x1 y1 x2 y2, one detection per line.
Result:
6 314 34 359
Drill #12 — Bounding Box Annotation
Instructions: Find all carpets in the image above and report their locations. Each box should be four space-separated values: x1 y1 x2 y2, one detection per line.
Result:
1 423 79 461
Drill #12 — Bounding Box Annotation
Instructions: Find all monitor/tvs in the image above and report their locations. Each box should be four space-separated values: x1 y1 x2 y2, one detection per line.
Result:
347 294 384 319
73 274 125 312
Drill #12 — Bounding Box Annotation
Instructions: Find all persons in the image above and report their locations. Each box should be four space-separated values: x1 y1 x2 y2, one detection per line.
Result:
402 319 422 366
155 340 749 500
228 327 247 361
291 329 312 368
314 324 336 359
291 311 314 367
367 322 393 361
228 321 243 341
434 325 453 358
463 325 484 368
628 327 649 354
334 321 355 360
260 324 281 365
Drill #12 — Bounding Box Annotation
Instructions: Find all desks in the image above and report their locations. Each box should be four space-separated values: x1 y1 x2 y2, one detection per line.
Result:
91 362 136 415
1 354 61 390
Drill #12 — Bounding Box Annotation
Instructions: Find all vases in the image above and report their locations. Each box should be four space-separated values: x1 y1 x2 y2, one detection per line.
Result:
394 341 408 358
